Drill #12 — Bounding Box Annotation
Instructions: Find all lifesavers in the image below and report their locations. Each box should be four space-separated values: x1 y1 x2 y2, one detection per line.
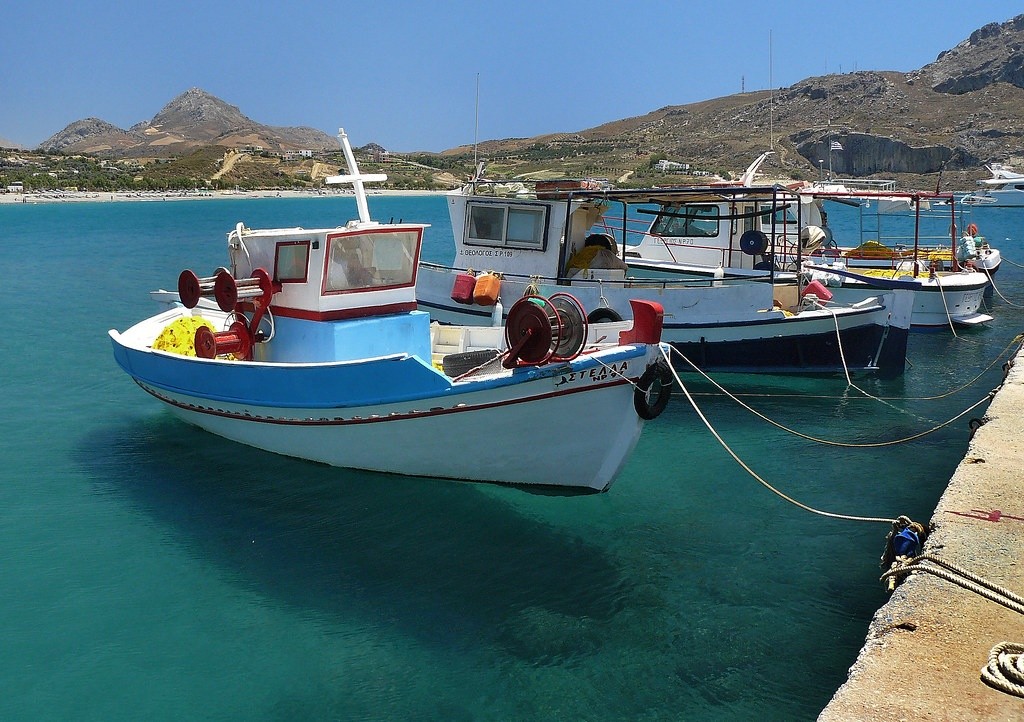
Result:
632 362 673 422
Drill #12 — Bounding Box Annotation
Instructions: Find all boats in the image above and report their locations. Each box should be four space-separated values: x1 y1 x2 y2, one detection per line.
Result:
755 179 1002 299
962 163 1024 207
414 160 923 376
597 152 988 331
108 129 675 496
